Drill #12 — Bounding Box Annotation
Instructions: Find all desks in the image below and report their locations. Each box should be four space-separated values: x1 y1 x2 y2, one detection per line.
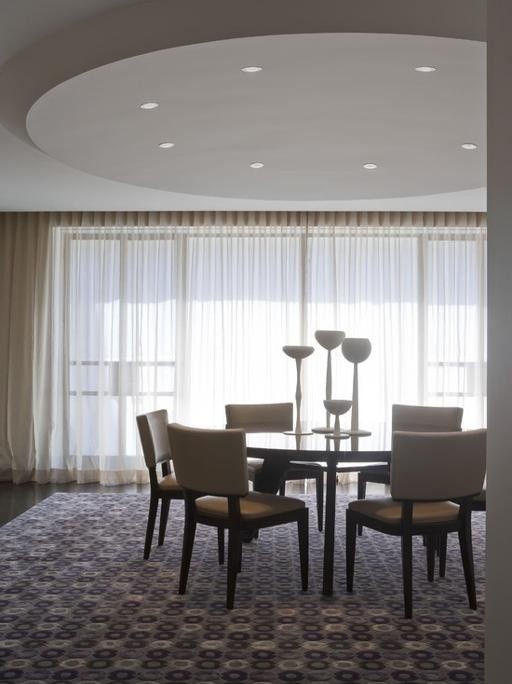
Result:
243 426 451 591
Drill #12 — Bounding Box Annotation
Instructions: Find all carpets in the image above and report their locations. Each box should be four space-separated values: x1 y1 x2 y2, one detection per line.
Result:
0 490 486 683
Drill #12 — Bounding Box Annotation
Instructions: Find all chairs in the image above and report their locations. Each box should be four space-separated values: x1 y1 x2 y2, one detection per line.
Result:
224 405 326 533
166 420 309 610
344 428 487 620
356 405 465 547
137 404 226 566
